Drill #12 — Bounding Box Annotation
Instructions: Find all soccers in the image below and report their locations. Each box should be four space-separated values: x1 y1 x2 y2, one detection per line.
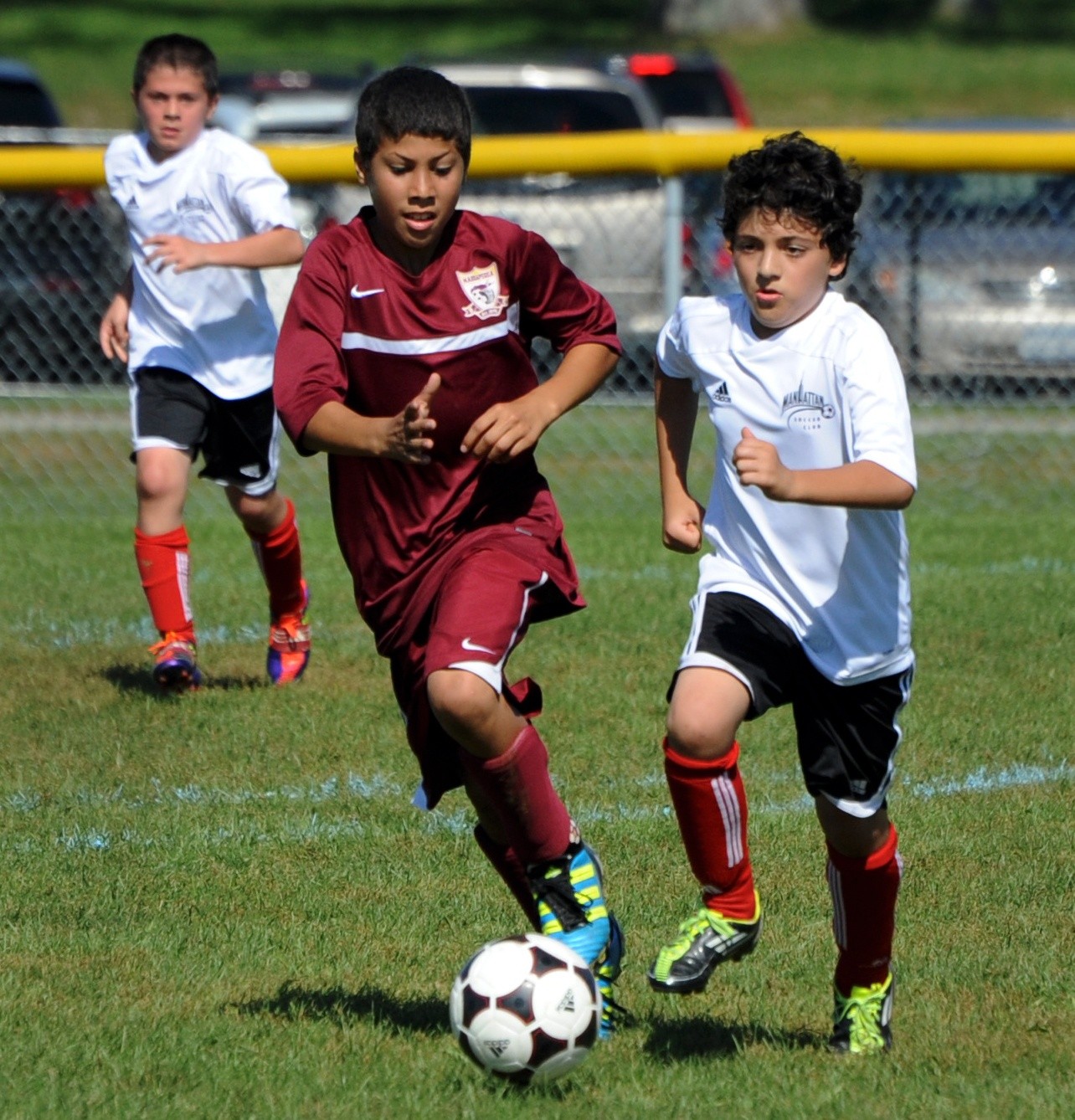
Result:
447 931 602 1086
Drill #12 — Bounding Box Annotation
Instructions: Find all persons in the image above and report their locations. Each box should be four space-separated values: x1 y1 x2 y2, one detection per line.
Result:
98 33 312 689
646 130 920 1056
272 66 626 1039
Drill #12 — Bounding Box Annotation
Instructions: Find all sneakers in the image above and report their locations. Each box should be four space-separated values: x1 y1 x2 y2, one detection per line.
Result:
267 580 311 686
152 643 200 692
646 888 763 995
590 914 627 1040
525 840 611 970
825 968 896 1055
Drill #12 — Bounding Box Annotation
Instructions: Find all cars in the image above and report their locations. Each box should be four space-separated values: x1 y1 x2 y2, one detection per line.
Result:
842 116 1074 389
206 27 759 357
0 64 129 386
